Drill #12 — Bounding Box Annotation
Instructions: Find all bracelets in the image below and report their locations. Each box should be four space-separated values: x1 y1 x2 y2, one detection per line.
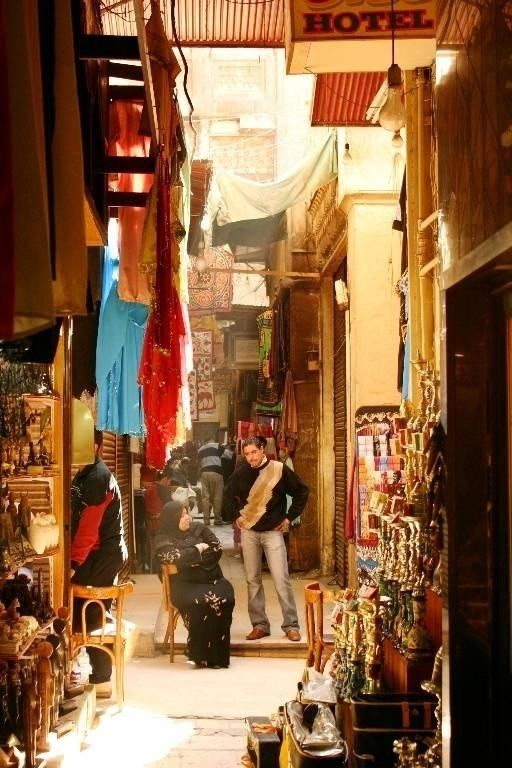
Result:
199 542 206 551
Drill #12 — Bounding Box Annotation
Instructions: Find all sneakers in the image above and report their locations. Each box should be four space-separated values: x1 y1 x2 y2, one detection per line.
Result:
122 620 141 663
81 681 112 698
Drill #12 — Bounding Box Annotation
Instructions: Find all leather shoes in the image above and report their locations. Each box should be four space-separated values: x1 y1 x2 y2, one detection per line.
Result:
246 628 270 640
286 629 301 641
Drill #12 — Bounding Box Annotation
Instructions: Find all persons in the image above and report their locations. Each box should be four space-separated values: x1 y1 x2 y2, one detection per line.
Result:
197 433 235 525
154 438 238 524
71 425 130 699
150 499 237 670
220 436 310 641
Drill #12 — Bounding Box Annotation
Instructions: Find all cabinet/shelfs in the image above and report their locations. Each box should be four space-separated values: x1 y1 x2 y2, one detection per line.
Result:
0 393 62 617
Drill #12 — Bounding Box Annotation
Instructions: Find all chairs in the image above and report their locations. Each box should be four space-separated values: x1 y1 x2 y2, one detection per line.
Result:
302 582 336 684
159 563 190 663
67 583 133 713
